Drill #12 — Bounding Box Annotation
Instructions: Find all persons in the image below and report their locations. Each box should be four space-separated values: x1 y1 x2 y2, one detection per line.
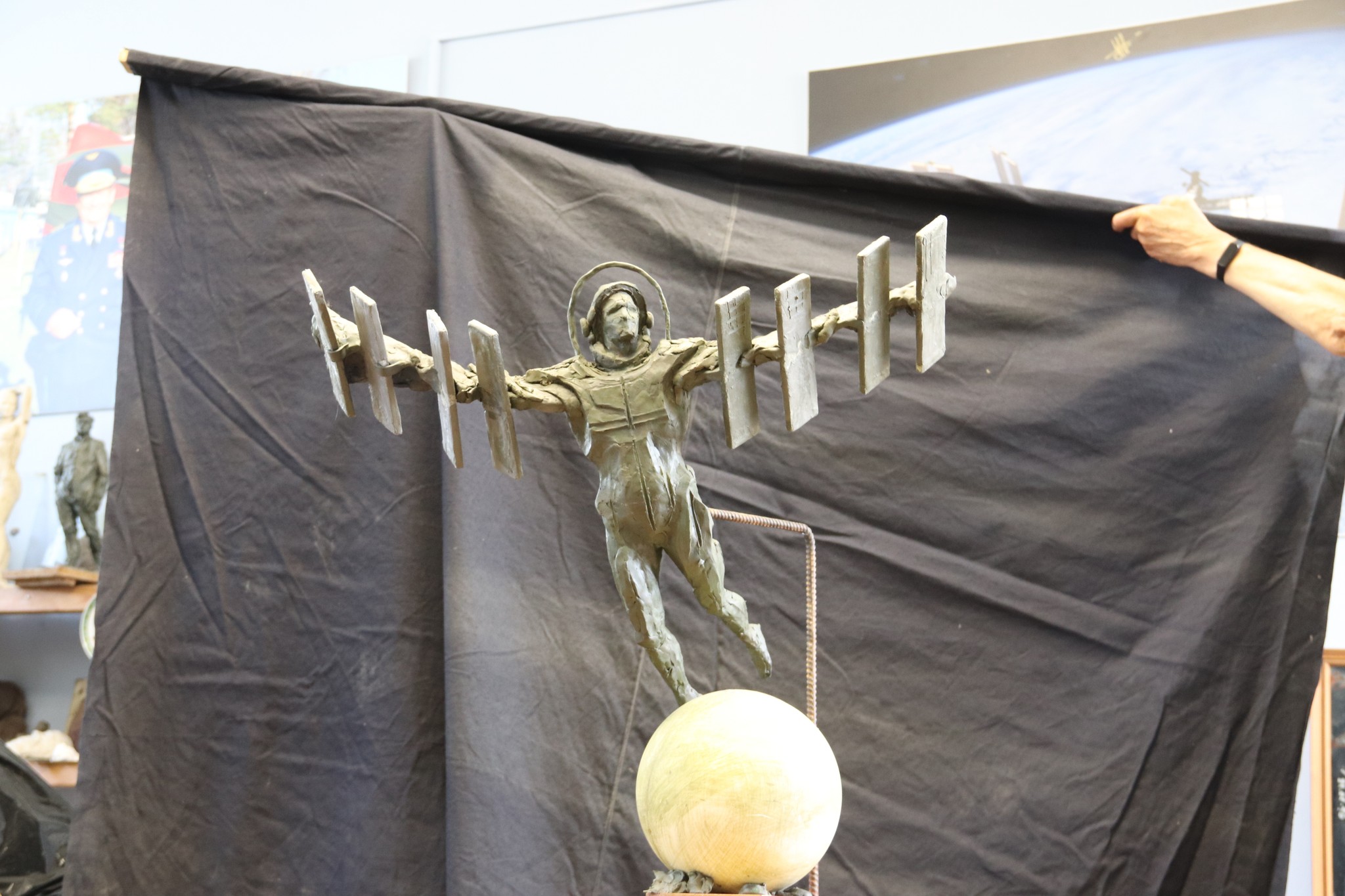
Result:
332 263 956 706
1110 194 1345 359
54 411 110 571
0 387 34 575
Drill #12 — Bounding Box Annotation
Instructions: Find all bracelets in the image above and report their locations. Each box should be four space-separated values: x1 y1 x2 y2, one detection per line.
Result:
1214 236 1245 282
